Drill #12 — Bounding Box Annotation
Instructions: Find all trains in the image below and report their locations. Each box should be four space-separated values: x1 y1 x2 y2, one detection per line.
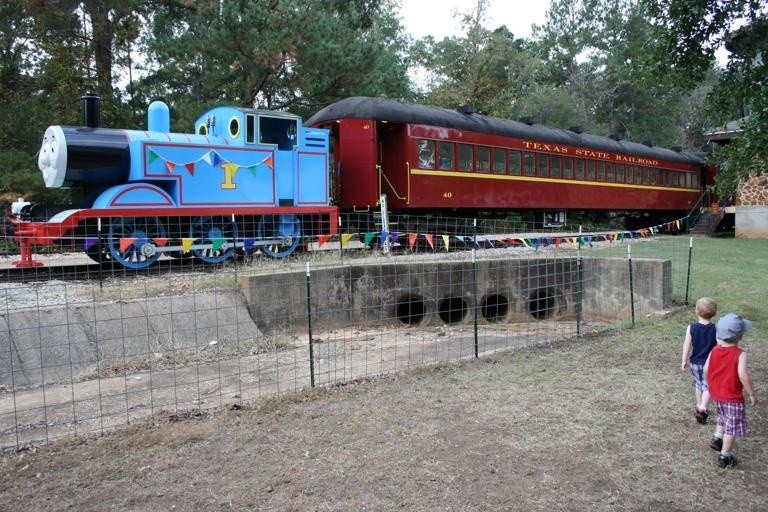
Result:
13 94 712 269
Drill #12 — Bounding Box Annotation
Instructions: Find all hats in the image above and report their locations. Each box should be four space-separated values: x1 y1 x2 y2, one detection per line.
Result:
714 313 753 340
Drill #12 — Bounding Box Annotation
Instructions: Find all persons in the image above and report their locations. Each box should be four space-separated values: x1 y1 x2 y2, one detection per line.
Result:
680 296 717 425
702 313 757 469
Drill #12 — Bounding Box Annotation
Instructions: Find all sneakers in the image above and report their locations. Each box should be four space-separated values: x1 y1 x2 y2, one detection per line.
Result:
710 436 722 451
717 454 737 467
693 406 708 424
